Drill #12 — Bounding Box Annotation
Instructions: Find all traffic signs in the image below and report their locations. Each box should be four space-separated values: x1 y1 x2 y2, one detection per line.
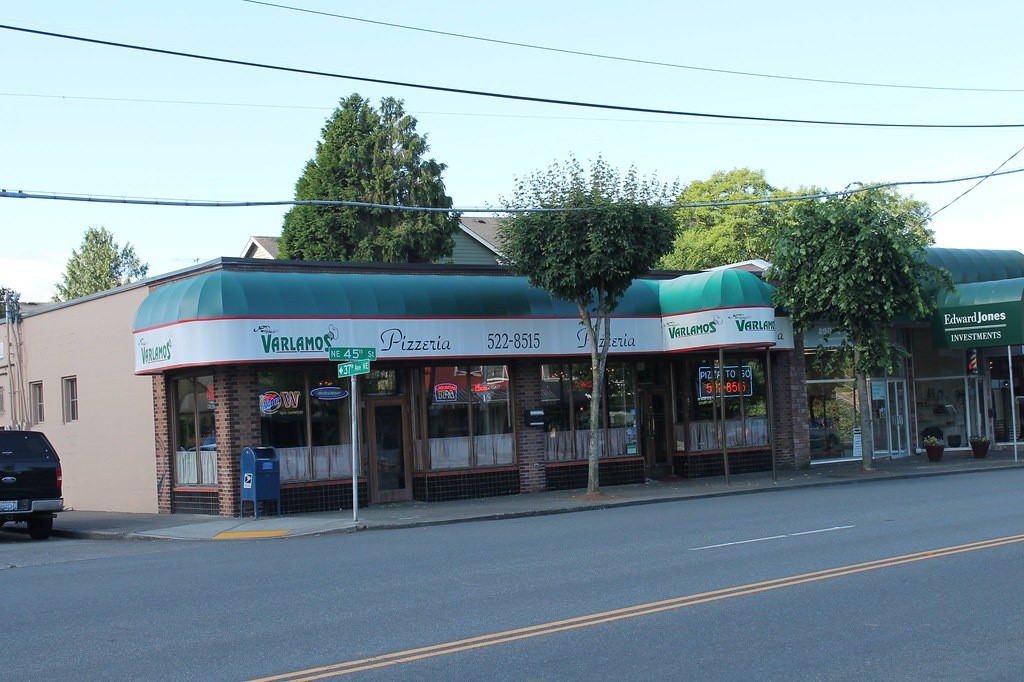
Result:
338 359 370 378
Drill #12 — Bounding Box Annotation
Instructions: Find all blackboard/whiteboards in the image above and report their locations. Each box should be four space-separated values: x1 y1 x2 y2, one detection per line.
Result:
853 433 862 457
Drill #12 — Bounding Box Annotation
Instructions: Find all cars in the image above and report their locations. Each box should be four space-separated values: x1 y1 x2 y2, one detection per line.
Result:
808 420 842 450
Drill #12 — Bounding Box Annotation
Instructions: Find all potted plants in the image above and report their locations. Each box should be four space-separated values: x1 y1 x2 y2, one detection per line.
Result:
969 435 991 459
923 436 946 461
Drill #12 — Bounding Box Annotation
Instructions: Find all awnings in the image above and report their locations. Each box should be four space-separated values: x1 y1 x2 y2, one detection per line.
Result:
133 249 1024 522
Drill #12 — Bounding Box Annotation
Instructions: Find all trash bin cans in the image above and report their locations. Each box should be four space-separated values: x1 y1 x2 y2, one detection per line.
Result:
240 446 280 518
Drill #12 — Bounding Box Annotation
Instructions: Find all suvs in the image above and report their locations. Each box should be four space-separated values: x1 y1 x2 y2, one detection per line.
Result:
0 430 65 539
